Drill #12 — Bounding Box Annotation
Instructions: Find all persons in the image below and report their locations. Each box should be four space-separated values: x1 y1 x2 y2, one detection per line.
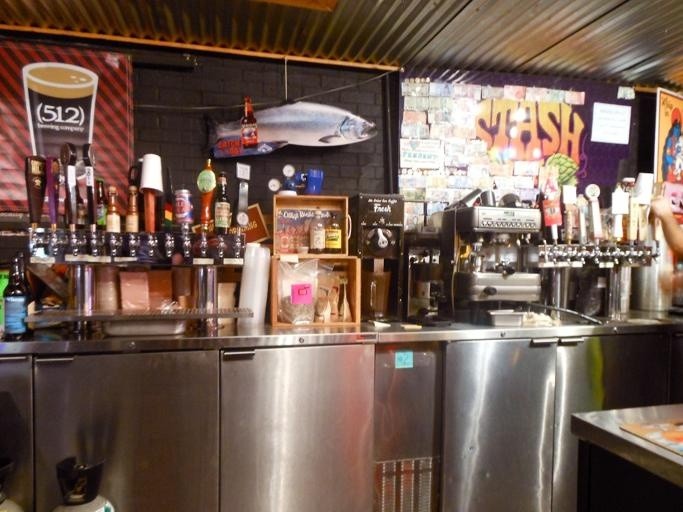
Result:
647 194 683 296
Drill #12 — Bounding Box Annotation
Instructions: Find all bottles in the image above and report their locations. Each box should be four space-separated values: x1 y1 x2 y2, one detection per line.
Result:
2 252 30 340
92 176 141 234
212 169 232 236
309 210 340 253
62 175 85 227
239 98 259 149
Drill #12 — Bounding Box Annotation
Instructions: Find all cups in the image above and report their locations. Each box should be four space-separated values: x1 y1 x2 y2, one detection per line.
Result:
136 153 165 198
304 169 325 194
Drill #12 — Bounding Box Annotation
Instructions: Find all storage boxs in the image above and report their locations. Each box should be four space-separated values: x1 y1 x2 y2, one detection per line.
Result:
271 193 363 331
